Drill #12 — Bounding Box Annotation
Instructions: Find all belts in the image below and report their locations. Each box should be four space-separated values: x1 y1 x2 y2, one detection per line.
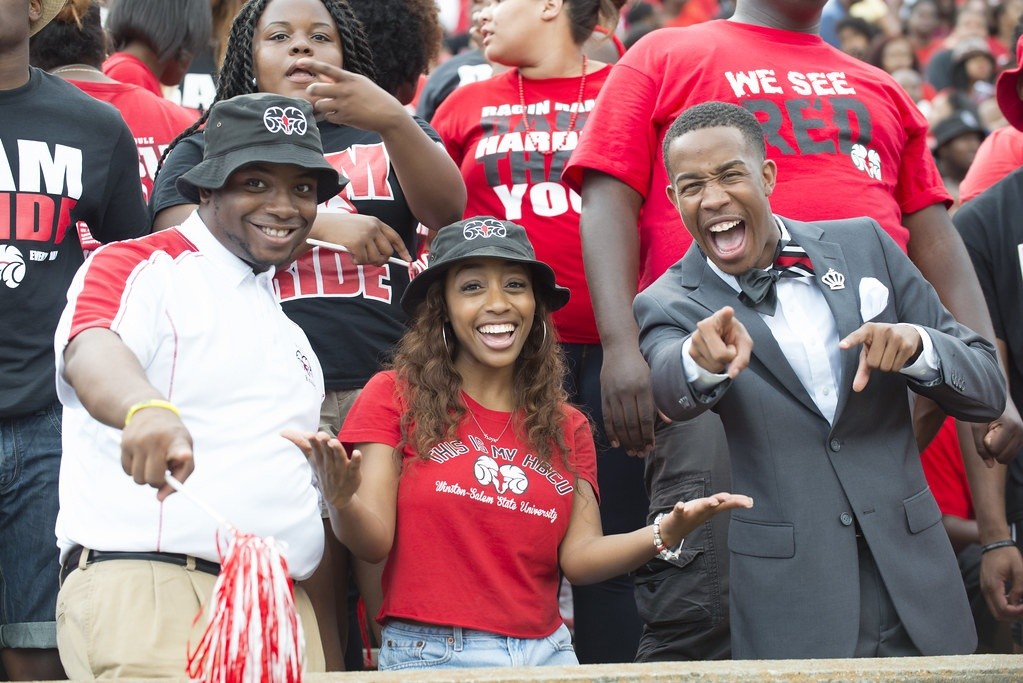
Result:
59 545 221 585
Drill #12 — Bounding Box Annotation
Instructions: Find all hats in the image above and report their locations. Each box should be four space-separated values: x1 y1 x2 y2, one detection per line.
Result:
175 92 350 205
29 0 68 38
995 34 1023 134
932 111 988 160
400 217 571 323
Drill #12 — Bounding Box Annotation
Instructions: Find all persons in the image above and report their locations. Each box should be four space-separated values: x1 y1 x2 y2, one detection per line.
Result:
633 98 1006 657
152 0 467 671
354 1 644 663
49 93 331 683
563 1 1023 663
0 0 246 683
282 213 757 670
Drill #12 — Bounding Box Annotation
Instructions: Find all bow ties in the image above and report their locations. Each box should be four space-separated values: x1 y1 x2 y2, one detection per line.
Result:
734 238 816 318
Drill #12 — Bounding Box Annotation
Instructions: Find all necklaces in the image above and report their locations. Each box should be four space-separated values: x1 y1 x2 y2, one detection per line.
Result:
517 55 587 150
461 390 517 444
51 68 104 76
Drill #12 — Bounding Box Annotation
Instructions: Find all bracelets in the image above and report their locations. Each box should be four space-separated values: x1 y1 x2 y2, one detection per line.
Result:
652 513 686 559
980 540 1017 553
124 401 179 430
